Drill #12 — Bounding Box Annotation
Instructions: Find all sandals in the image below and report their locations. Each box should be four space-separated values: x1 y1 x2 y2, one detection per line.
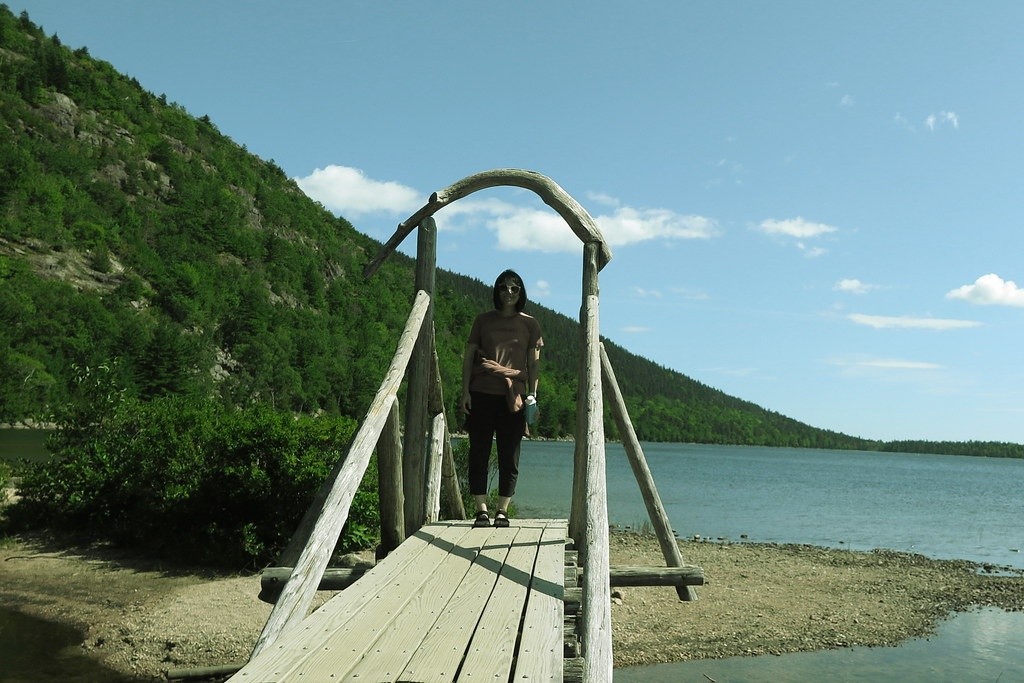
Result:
493 510 510 527
474 511 490 526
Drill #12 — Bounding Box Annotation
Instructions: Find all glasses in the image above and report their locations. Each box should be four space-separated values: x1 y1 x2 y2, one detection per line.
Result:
499 285 521 294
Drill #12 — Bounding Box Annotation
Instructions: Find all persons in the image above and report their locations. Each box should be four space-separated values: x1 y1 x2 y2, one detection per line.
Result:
459 270 543 527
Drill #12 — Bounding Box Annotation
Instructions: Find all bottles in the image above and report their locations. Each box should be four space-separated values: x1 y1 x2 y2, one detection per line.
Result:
524 395 537 425
